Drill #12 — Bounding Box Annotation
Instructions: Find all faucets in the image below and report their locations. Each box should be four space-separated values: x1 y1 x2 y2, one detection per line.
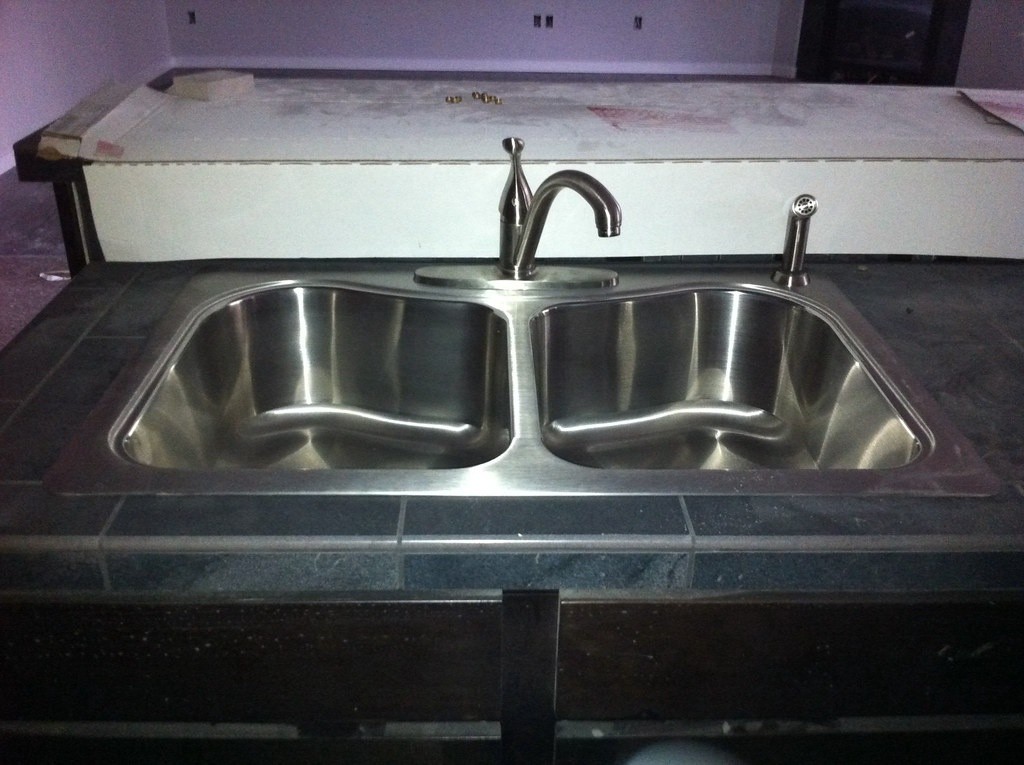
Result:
496 136 625 277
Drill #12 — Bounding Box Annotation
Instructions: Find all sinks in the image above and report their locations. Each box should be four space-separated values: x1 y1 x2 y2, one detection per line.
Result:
80 278 520 493
526 275 1007 495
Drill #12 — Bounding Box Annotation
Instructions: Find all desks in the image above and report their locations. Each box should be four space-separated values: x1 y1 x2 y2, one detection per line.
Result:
17 78 1024 269
